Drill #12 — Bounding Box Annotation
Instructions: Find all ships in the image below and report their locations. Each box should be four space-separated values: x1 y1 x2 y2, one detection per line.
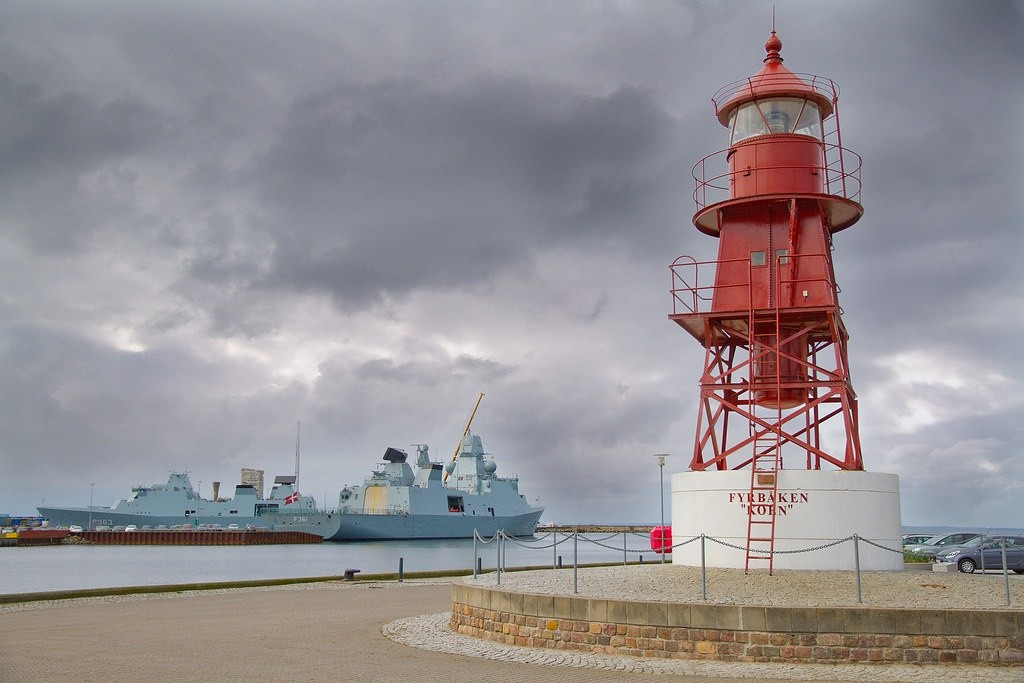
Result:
323 391 546 541
34 421 343 541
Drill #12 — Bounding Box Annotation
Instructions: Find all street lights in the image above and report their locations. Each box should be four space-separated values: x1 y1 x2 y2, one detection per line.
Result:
652 453 670 563
88 483 96 532
196 480 202 527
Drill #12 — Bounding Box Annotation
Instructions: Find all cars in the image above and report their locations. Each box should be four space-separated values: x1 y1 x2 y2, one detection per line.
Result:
0 521 258 533
935 533 1024 575
910 531 994 565
902 533 944 554
